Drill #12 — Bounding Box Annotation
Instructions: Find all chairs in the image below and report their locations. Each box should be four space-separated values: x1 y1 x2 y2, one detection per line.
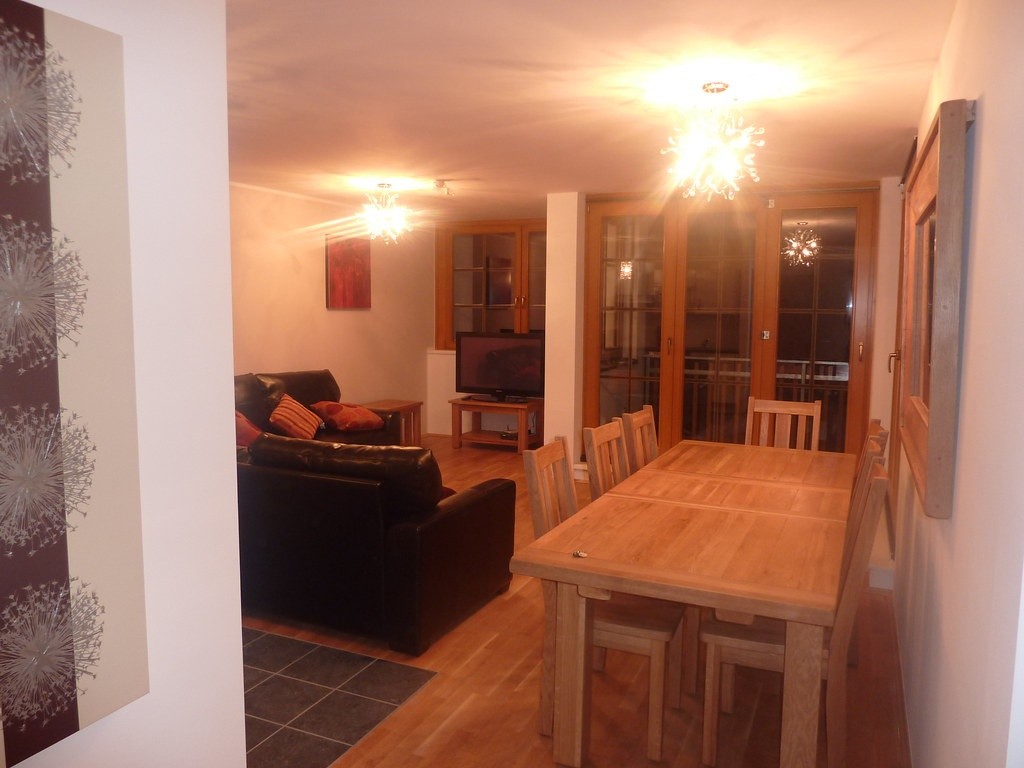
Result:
525 435 702 760
622 401 662 472
743 396 824 454
585 418 633 494
701 459 892 767
839 417 891 615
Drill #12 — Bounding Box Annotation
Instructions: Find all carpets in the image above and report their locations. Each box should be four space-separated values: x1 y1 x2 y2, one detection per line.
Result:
241 625 438 768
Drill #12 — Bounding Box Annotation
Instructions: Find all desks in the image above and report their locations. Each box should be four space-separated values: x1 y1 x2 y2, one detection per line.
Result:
508 437 858 768
448 389 544 455
362 397 426 449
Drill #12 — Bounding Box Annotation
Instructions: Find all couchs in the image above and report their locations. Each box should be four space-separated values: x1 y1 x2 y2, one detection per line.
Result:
235 367 520 656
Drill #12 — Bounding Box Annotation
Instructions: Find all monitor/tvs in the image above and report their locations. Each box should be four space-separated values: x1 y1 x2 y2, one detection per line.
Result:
456 332 546 396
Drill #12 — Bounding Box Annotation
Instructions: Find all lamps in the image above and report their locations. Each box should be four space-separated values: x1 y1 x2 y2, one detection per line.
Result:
359 182 416 246
784 221 821 270
618 257 633 279
664 80 768 204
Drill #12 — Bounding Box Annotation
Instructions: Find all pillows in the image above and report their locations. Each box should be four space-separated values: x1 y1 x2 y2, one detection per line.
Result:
310 398 386 433
269 391 324 442
